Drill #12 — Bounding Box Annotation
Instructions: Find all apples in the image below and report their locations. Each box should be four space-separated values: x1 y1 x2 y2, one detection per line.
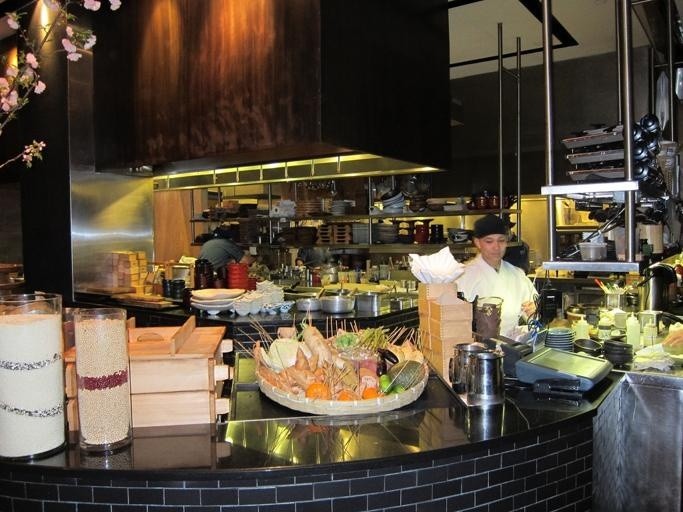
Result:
379 375 405 395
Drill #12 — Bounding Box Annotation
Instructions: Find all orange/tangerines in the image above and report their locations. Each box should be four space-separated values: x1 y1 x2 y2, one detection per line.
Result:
337 390 361 401
362 387 383 400
306 384 331 401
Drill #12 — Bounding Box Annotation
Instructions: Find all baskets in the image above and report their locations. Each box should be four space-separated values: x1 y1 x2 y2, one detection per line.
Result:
256 341 428 416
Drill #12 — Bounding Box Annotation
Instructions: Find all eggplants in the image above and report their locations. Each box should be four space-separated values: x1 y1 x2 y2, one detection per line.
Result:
378 348 399 364
377 358 387 377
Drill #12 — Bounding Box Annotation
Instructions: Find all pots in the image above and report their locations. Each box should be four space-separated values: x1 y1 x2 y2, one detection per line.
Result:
353 288 379 313
320 281 358 313
578 241 607 262
295 286 326 312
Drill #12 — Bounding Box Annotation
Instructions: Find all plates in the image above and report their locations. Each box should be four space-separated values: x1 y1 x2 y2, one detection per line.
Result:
188 288 246 313
294 198 356 217
544 327 576 352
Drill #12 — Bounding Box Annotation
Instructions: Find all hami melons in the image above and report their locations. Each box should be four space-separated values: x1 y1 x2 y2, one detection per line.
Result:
389 361 425 387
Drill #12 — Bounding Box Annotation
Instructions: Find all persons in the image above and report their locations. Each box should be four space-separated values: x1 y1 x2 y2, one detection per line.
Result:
448 212 541 339
196 229 249 285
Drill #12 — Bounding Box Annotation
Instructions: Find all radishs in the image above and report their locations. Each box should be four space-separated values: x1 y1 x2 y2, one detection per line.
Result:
304 327 332 363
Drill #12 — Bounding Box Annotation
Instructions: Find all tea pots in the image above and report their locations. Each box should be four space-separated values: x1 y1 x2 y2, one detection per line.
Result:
448 342 504 406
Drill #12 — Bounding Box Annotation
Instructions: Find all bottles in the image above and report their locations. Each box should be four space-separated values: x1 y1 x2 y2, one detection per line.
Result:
625 311 640 347
641 313 658 347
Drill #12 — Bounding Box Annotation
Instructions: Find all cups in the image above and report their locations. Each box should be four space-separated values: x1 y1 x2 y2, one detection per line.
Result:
587 322 599 341
0 291 68 462
72 304 134 455
487 196 499 208
474 196 488 209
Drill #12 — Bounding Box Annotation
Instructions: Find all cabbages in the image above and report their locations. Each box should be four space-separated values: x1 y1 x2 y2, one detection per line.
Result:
266 339 311 371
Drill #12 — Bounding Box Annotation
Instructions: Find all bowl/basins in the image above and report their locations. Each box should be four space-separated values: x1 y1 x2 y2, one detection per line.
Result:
233 301 295 316
575 338 601 356
399 223 469 244
160 257 256 295
380 190 404 214
375 222 399 243
603 340 633 367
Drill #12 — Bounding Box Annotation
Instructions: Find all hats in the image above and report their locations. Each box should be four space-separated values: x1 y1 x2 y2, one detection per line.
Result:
473 215 506 238
